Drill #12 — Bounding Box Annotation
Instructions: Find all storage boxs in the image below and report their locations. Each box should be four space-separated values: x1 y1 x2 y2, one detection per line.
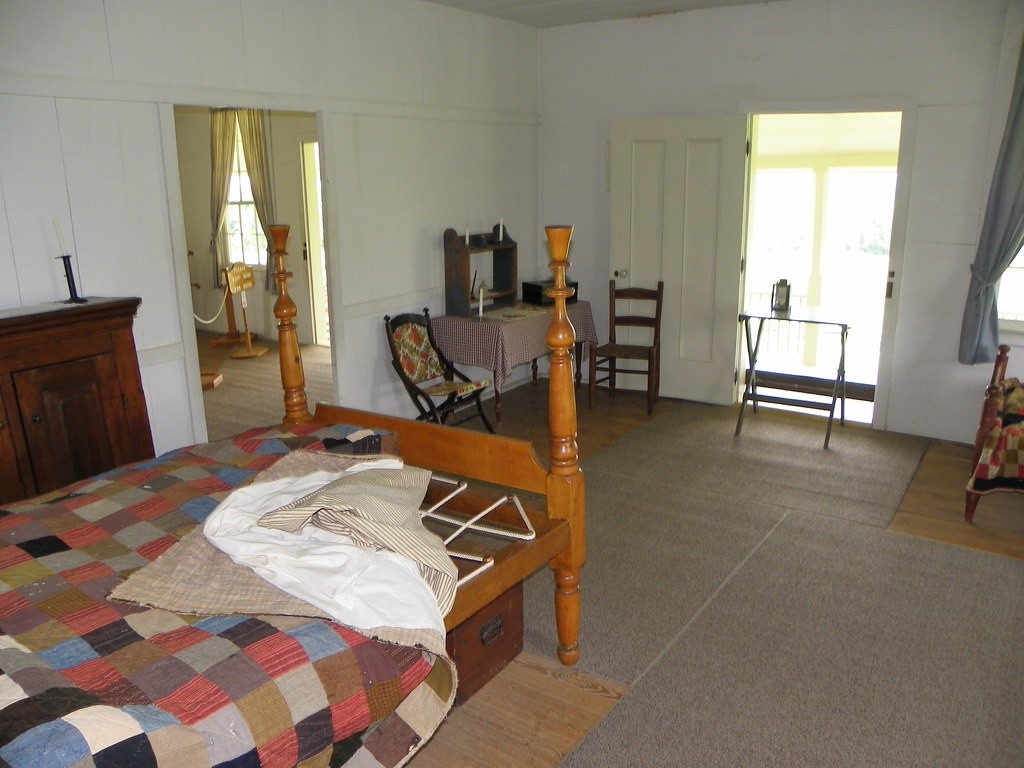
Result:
522 280 578 306
428 528 525 708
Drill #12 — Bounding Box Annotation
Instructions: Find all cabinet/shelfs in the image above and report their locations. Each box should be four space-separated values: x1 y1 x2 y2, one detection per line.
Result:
0 296 156 506
444 224 517 318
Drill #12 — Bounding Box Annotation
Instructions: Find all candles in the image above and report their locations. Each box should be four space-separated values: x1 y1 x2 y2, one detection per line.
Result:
53 218 68 256
465 229 469 245
479 287 483 317
499 217 503 241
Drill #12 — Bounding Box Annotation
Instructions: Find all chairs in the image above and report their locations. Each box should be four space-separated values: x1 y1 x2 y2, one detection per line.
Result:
589 280 664 416
384 307 497 434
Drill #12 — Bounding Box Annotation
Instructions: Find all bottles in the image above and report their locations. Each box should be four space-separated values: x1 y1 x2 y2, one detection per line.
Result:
771 279 791 310
479 280 488 294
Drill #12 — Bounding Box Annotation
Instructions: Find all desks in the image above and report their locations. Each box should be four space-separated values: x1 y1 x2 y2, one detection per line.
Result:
734 303 858 449
431 299 599 432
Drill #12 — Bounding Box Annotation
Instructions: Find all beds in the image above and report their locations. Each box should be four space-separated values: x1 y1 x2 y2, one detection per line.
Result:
0 225 587 768
966 345 1024 523
